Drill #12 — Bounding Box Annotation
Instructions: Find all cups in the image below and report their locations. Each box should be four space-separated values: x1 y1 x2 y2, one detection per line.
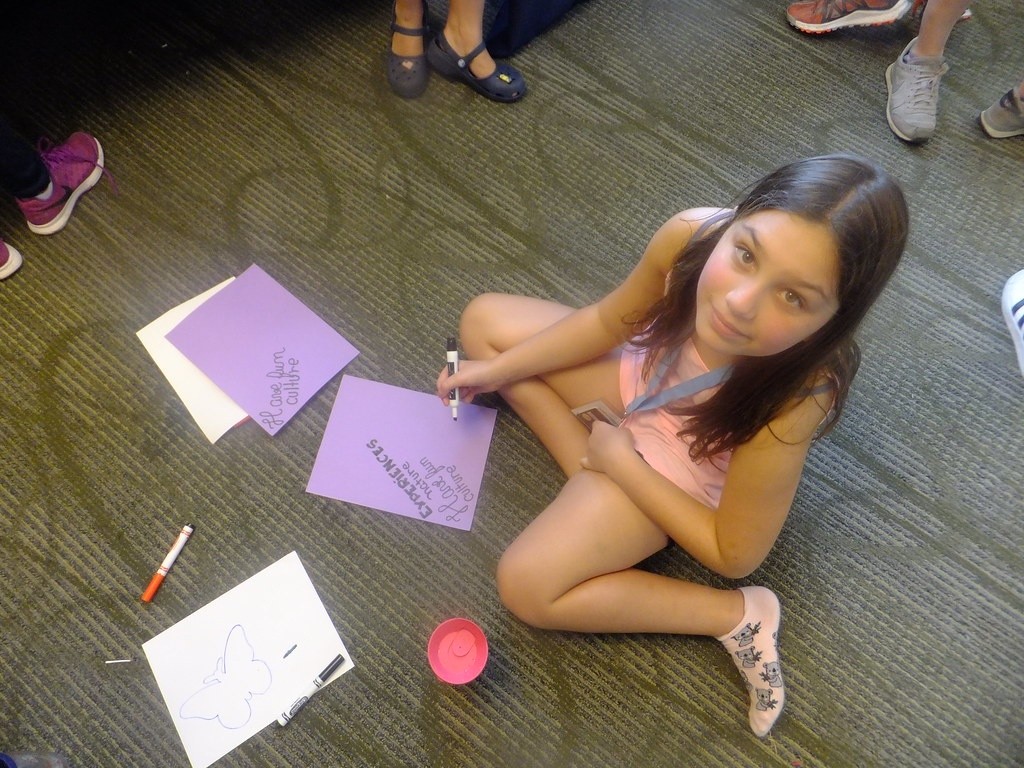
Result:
427 618 488 685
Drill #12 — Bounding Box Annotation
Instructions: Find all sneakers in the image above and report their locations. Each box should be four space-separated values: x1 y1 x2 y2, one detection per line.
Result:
0 240 23 280
980 87 1024 138
16 132 117 235
786 0 912 34
1002 267 1024 378
885 36 949 143
919 0 972 21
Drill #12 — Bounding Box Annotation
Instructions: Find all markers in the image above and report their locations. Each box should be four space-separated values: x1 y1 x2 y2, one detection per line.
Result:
140 522 196 604
445 337 460 422
276 654 346 728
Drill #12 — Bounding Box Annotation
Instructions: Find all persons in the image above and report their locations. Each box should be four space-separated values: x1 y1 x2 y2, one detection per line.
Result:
784 0 1024 146
437 151 908 738
385 0 526 103
0 117 119 282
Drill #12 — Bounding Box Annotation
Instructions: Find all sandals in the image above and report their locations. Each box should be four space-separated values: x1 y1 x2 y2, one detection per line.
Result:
425 32 525 102
387 0 430 98
